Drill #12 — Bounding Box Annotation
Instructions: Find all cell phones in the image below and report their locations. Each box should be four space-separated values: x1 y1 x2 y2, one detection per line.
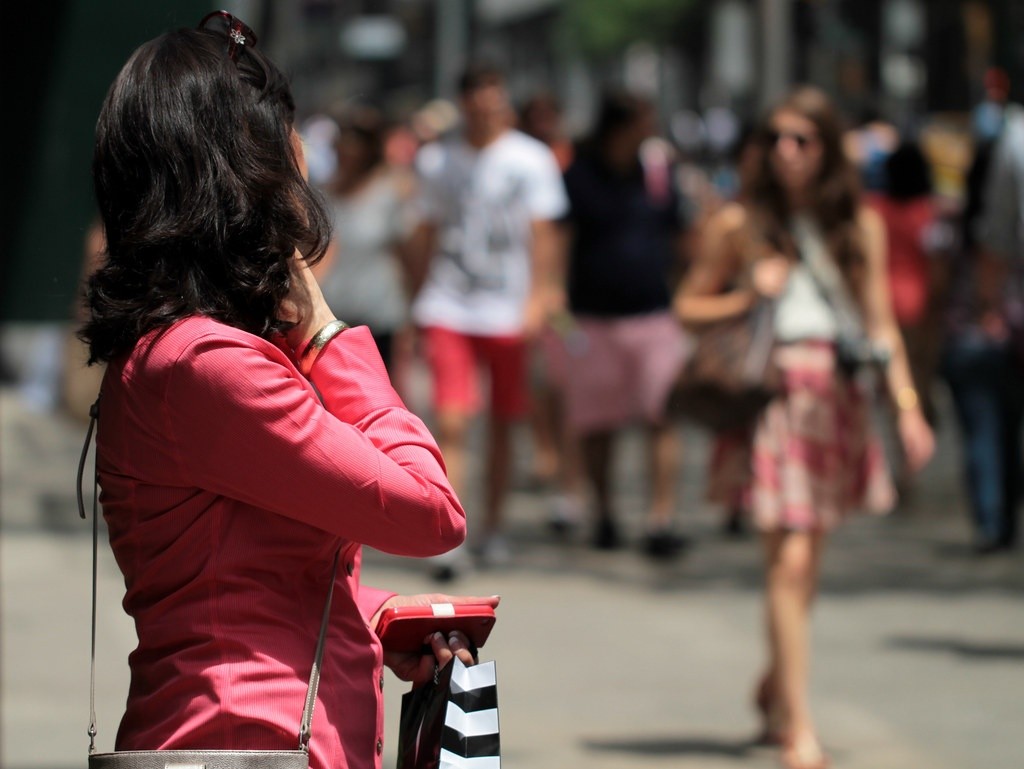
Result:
375 603 496 653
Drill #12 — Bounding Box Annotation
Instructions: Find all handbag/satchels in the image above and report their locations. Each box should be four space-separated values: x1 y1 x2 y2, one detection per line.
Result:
89 749 311 769
396 654 502 768
680 317 785 436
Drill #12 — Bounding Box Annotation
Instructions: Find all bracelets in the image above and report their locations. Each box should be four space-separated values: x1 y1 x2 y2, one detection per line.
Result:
296 321 349 381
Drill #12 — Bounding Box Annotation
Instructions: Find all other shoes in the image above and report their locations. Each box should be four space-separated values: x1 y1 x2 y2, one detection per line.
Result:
426 533 506 581
592 515 685 558
755 672 827 769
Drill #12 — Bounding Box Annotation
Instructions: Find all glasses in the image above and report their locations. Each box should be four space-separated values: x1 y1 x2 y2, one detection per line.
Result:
199 10 257 59
763 132 820 149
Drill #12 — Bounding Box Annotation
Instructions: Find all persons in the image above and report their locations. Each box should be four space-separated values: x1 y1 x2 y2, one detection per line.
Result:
280 57 1023 586
670 85 938 769
400 64 572 580
559 77 712 563
74 7 503 769
